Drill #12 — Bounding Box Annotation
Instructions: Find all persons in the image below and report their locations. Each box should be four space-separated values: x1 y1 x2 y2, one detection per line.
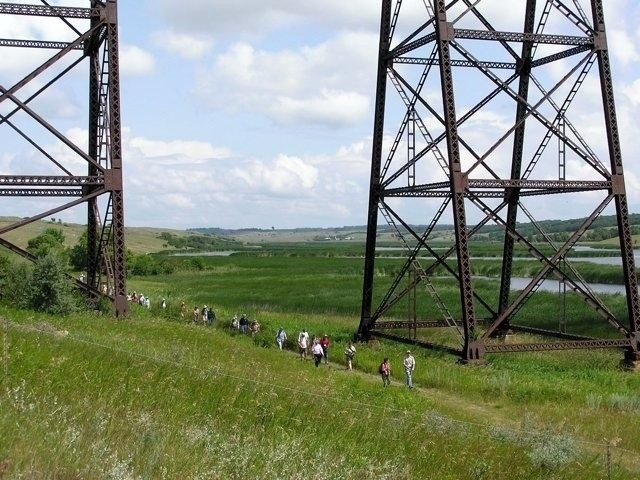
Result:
403 350 415 389
160 299 165 309
229 314 260 337
346 342 356 371
276 327 287 350
193 307 215 326
298 329 330 367
180 302 187 318
382 358 391 387
77 272 150 309
